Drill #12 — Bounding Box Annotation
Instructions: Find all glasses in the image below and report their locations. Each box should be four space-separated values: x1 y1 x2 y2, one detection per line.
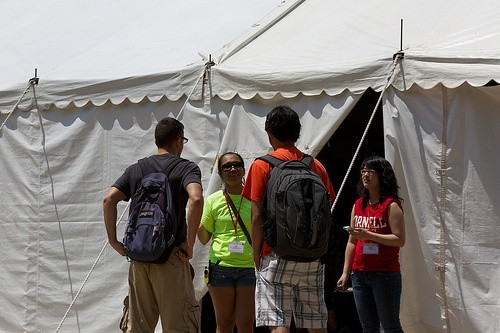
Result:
361 169 376 176
177 136 188 144
220 163 244 172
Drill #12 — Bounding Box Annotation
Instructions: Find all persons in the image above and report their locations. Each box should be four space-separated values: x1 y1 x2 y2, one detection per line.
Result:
337 157 406 333
241 106 336 333
102 117 204 333
196 152 263 333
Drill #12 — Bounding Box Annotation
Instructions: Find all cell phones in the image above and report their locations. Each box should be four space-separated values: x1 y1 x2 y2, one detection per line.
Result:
343 226 355 233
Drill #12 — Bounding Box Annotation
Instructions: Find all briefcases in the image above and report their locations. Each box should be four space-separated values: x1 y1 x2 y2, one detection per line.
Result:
327 287 363 333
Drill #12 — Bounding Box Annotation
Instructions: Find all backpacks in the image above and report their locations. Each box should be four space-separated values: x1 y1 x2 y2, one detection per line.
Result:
254 155 331 263
123 156 189 264
